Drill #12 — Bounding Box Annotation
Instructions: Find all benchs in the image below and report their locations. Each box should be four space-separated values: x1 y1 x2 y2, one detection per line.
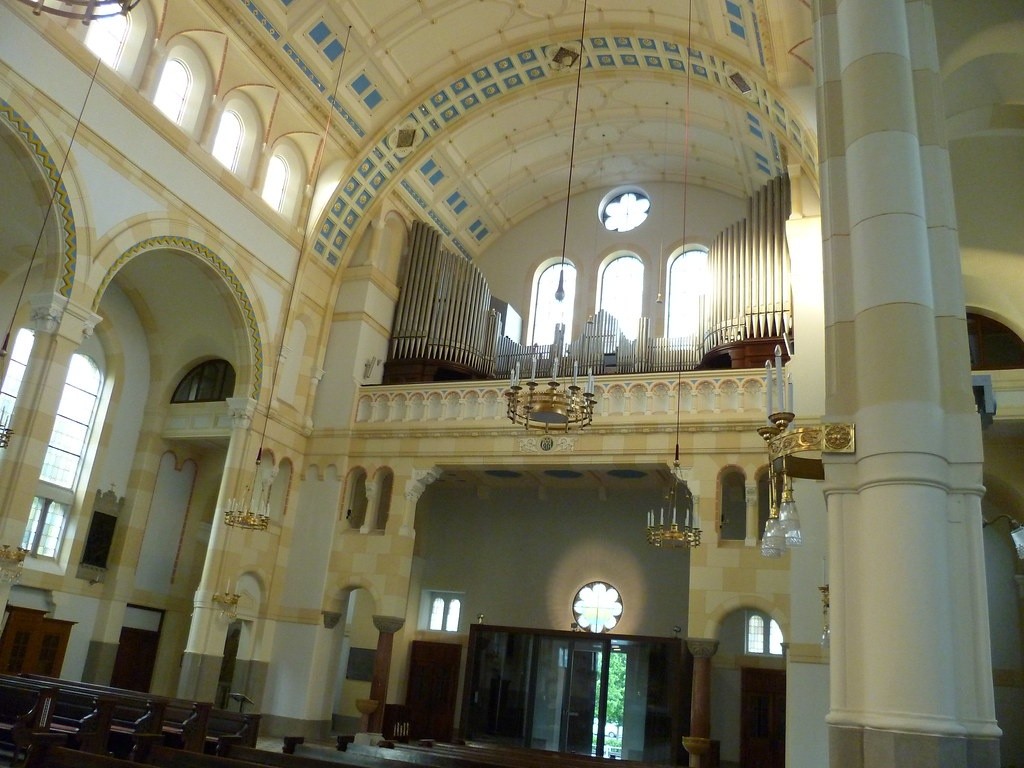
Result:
23 725 685 768
0 673 261 768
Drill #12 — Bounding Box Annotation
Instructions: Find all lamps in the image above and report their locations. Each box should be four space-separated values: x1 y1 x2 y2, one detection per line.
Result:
212 577 242 624
818 556 829 650
644 0 702 548
597 155 653 233
587 133 606 324
982 513 1024 560
504 0 597 433
224 25 352 532
655 102 670 303
555 0 587 302
18 0 140 26
757 344 856 560
0 58 102 450
90 576 99 585
0 535 29 585
